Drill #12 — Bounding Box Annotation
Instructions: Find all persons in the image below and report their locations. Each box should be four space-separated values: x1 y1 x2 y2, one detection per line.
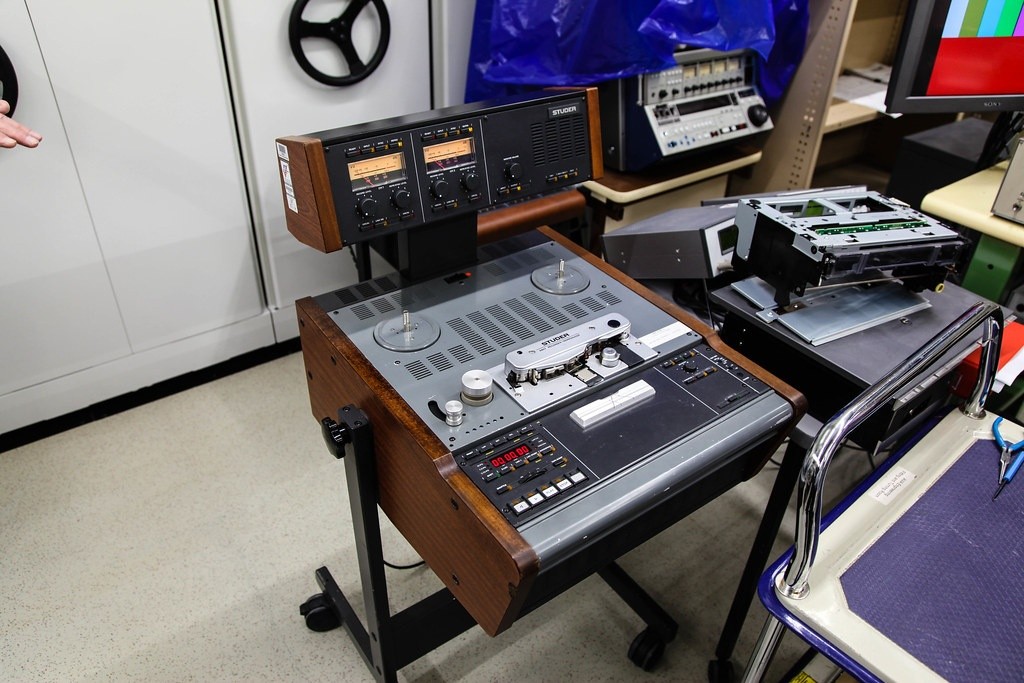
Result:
1 99 42 149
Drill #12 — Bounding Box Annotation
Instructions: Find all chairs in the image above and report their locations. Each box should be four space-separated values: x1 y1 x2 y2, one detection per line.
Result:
738 302 1024 683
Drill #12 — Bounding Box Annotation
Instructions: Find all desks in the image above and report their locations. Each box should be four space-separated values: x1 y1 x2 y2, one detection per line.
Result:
581 138 764 266
921 161 1024 306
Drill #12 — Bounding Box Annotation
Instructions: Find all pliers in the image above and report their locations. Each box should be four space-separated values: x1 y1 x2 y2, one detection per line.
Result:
992 416 1024 484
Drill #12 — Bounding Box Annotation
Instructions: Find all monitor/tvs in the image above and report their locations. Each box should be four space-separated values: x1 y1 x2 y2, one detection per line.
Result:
884 0 1024 113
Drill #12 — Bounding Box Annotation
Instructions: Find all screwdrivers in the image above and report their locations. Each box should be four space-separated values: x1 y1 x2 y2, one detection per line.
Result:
992 452 1024 501
